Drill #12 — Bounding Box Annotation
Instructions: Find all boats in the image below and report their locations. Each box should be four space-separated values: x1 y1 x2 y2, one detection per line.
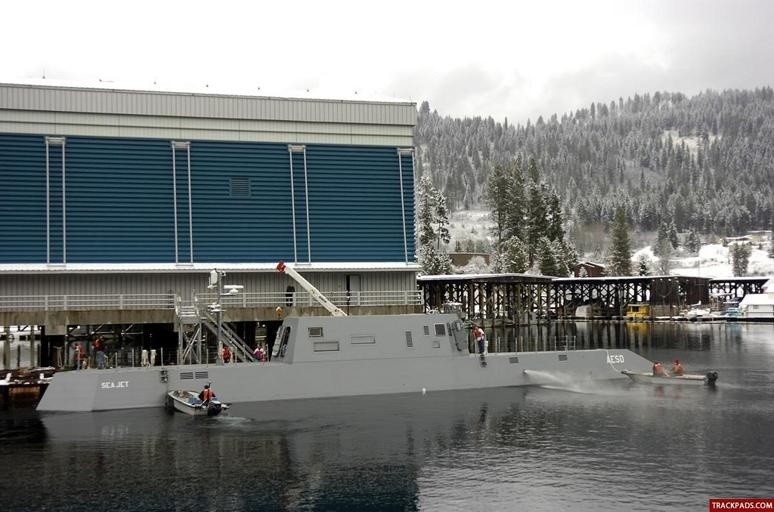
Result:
655 293 774 321
167 388 232 416
621 369 719 386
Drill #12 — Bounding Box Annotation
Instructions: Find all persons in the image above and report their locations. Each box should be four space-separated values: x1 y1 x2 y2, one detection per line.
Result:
653 360 684 378
223 345 231 363
199 385 216 402
474 326 486 354
252 343 266 362
73 335 114 369
285 283 295 307
